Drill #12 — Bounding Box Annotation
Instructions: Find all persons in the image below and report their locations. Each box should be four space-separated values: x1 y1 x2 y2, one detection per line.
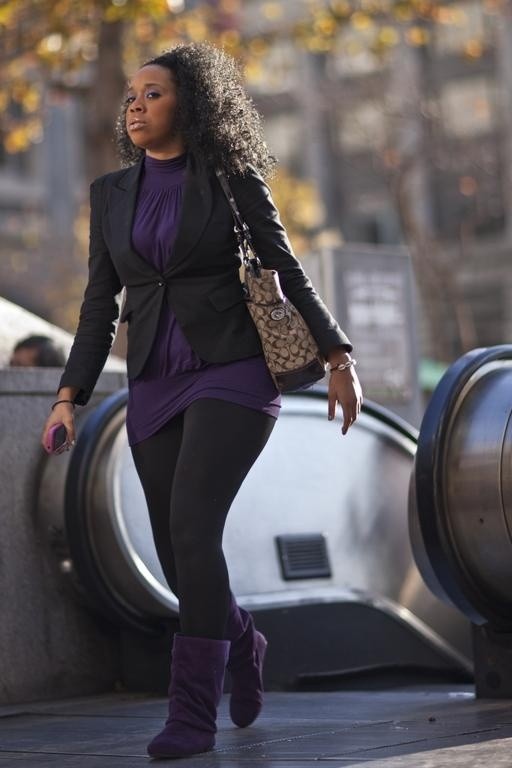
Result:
9 333 66 366
41 41 363 759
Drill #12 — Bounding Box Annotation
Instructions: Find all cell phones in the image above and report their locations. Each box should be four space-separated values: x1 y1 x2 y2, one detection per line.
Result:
45 424 67 455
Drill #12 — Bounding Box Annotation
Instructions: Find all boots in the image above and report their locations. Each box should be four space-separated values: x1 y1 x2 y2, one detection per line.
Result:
228 588 268 728
147 630 231 759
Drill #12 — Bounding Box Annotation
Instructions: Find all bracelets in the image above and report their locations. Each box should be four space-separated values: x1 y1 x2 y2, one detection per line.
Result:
330 359 356 372
51 400 76 410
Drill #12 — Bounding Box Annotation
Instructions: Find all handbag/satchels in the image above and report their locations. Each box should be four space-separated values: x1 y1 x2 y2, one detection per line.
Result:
244 263 326 397
